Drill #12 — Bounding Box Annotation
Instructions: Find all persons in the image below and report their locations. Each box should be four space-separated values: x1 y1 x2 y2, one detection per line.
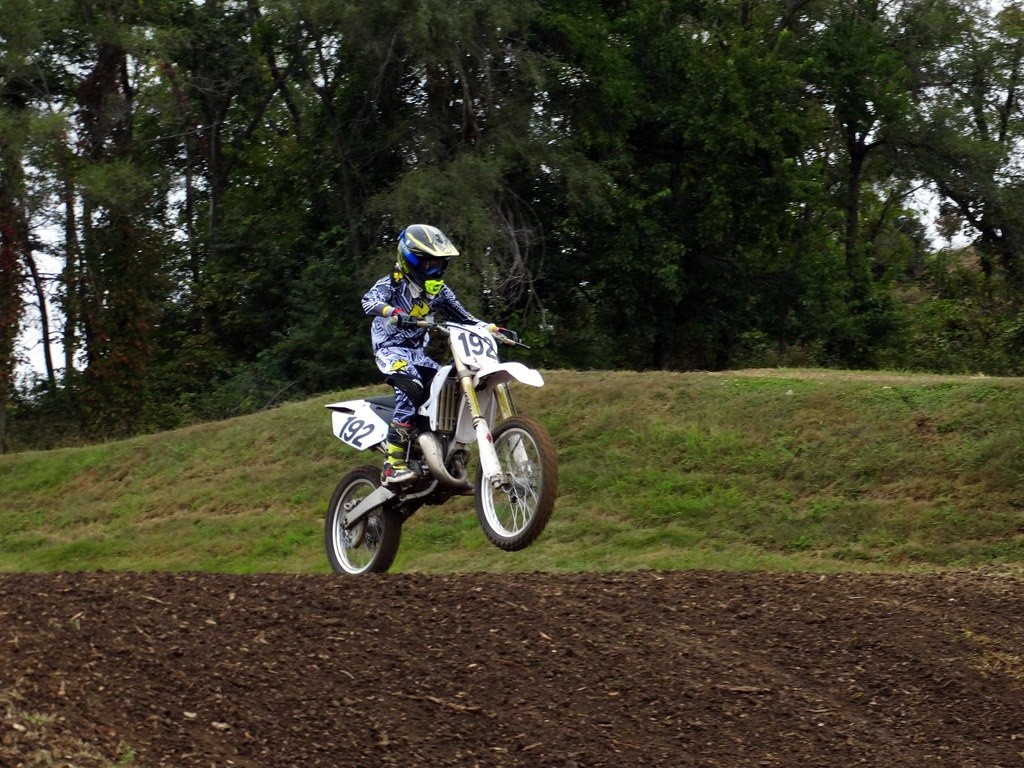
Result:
360 225 517 488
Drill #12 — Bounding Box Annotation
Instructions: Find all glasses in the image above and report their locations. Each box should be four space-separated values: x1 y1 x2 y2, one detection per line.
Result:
415 254 450 275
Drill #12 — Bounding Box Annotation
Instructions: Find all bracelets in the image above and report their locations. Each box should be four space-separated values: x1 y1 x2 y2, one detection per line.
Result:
387 307 396 316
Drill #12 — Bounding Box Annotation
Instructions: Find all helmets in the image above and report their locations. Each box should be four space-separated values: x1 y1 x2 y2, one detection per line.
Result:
397 223 460 294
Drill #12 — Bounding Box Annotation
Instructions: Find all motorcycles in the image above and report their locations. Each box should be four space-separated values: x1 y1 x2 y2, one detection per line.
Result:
324 316 560 579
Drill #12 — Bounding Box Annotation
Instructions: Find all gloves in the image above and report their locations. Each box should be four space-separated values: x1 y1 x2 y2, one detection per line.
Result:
498 327 519 347
392 305 414 330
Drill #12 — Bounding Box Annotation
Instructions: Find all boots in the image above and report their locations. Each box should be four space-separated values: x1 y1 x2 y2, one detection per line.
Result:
381 422 419 487
456 481 474 496
